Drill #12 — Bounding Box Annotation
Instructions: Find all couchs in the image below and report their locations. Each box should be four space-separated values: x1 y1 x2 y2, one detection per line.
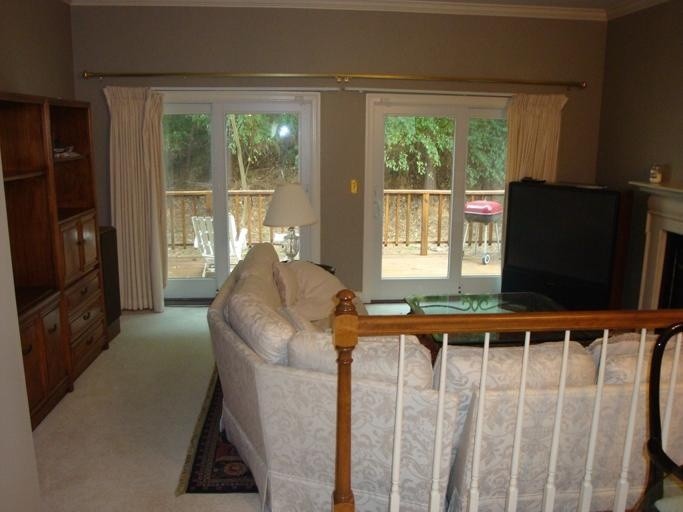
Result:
207 259 683 511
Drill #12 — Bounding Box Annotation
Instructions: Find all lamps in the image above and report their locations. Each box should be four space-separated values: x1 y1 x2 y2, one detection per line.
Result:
263 183 319 262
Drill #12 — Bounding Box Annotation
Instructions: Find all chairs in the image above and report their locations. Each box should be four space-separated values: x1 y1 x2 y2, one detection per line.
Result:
192 213 248 278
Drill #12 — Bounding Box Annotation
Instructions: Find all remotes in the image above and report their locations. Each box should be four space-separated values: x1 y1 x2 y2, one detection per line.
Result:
522 179 546 183
576 184 607 189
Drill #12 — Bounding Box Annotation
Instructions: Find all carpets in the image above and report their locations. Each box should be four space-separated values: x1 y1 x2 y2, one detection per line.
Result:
174 362 260 497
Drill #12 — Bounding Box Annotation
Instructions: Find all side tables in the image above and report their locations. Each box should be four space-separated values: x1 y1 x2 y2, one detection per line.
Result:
281 260 336 277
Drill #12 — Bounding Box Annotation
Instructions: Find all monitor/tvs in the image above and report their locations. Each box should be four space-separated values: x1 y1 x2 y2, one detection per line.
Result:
504 181 634 289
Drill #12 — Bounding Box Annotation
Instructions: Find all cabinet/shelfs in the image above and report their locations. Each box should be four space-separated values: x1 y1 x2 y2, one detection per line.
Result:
500 181 634 309
0 91 110 432
